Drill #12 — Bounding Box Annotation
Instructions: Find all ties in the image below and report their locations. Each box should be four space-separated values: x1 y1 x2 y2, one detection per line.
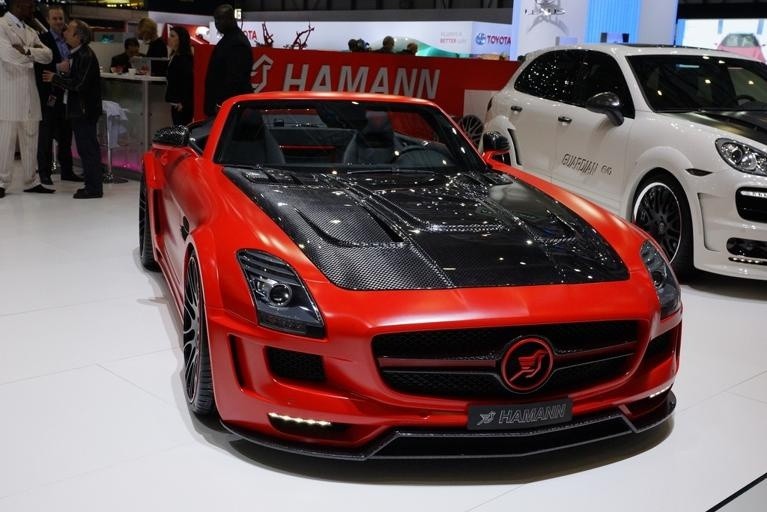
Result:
57 37 69 60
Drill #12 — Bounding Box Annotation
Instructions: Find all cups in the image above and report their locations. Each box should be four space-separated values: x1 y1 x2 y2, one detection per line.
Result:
127 69 136 77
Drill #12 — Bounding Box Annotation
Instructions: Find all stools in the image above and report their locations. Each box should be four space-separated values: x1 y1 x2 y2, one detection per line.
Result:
94 110 129 184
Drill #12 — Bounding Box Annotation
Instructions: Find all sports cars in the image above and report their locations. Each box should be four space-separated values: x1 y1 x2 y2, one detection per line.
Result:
136 91 684 464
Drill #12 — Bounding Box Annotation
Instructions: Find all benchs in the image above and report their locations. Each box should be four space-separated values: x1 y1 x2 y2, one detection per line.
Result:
269 127 353 162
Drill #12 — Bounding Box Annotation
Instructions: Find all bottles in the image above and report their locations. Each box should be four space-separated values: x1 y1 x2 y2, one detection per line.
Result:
99 65 103 76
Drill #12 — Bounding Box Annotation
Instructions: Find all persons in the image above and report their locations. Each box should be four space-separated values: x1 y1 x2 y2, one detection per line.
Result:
0 0 56 198
137 17 168 77
42 19 103 199
203 4 254 118
164 26 194 127
348 36 417 57
109 38 145 74
33 5 85 185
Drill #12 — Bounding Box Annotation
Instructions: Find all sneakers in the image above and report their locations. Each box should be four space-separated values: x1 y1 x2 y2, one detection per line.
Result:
71 185 104 199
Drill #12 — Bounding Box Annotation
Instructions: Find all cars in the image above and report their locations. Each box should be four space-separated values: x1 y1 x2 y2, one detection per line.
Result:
477 44 766 283
712 33 766 63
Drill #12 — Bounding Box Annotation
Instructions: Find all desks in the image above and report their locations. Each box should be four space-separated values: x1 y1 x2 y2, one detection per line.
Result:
51 71 174 181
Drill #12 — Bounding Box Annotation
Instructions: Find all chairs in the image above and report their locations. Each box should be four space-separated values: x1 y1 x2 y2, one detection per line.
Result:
343 111 402 164
219 109 285 164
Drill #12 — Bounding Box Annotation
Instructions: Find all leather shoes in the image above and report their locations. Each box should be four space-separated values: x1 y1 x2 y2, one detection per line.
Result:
22 183 57 194
39 175 55 186
59 173 86 184
